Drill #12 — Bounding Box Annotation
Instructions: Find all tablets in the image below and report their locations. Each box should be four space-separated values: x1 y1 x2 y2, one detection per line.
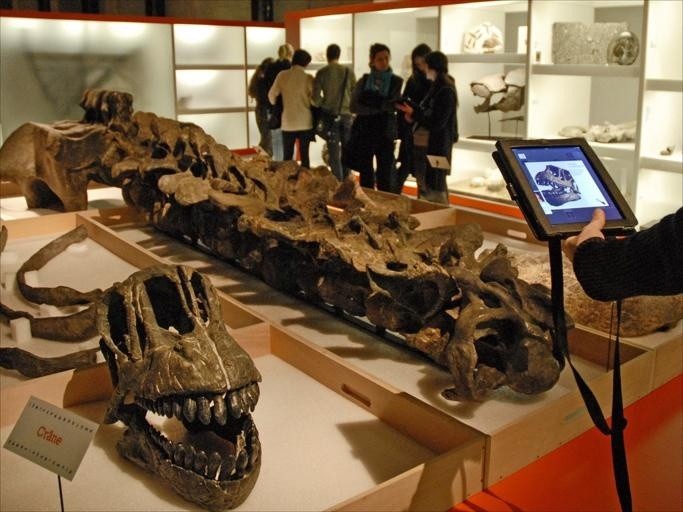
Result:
492 136 639 241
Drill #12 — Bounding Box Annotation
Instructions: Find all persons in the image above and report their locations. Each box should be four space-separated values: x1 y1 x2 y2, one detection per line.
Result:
560 204 683 302
310 43 358 182
349 42 405 189
246 57 273 159
267 48 314 169
260 42 298 160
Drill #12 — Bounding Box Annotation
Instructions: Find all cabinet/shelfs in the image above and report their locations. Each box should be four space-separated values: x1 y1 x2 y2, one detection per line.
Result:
353 7 442 194
440 0 529 214
173 18 285 159
530 0 644 233
299 7 353 180
635 5 682 236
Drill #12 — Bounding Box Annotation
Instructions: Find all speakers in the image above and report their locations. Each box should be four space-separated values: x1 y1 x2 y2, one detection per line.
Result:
251 0 274 22
0 0 13 8
145 0 165 16
81 0 99 13
38 0 50 12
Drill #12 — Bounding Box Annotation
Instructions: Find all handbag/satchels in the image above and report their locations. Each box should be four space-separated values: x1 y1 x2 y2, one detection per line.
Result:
311 107 337 140
268 108 280 129
